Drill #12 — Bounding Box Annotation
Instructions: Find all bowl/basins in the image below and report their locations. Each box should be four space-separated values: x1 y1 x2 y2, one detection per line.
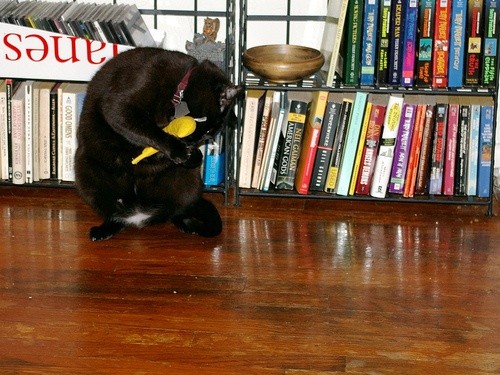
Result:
242 44 325 83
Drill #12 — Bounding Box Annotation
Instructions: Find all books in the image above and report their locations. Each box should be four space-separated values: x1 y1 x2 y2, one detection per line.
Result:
204 124 227 186
238 90 494 198
0 79 87 185
316 0 500 88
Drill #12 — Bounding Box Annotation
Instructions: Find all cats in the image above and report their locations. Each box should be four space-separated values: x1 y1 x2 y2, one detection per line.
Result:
74 46 247 242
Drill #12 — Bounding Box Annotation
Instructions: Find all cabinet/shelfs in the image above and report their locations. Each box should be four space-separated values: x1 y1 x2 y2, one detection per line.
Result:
0 1 235 206
232 1 499 216
